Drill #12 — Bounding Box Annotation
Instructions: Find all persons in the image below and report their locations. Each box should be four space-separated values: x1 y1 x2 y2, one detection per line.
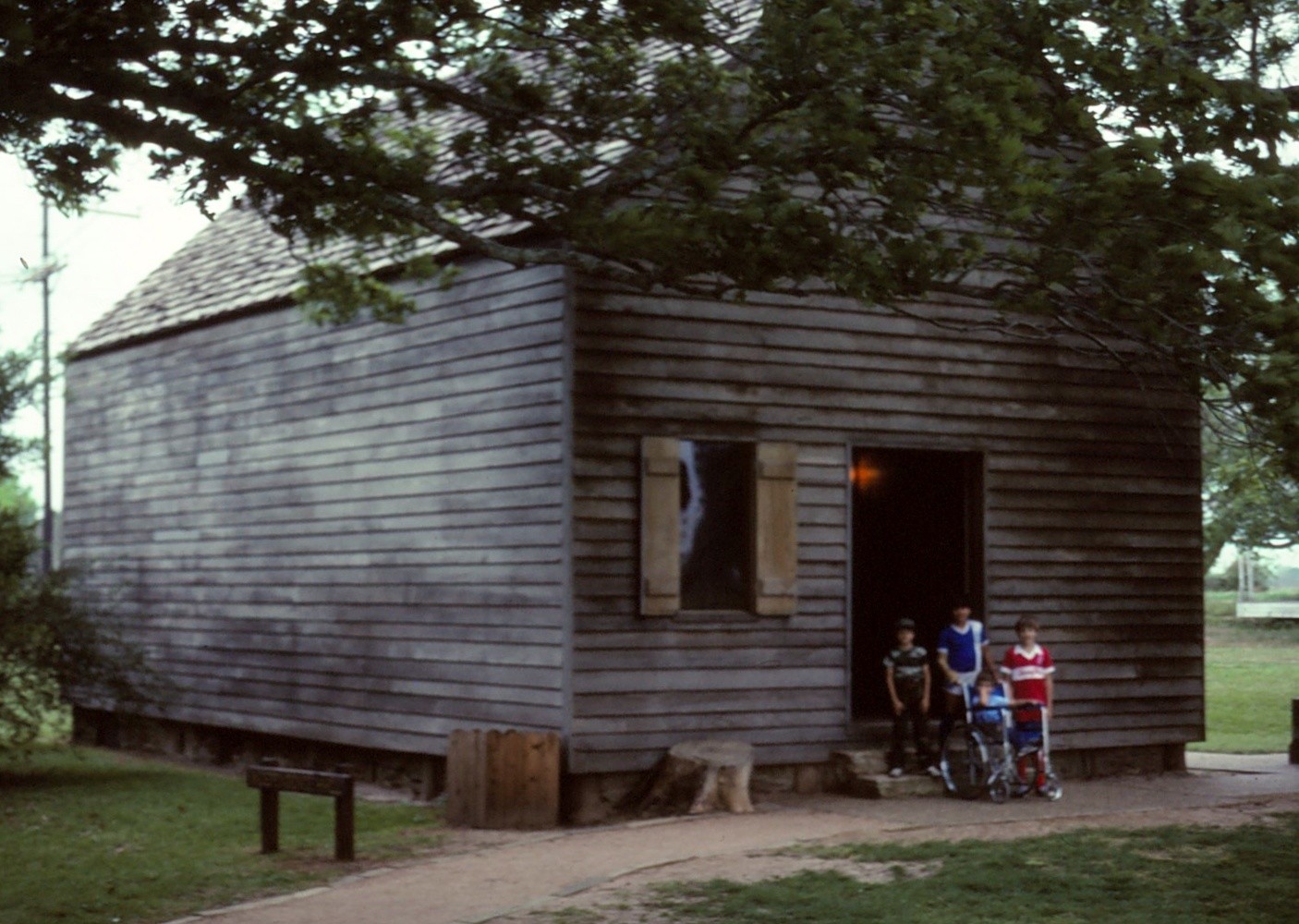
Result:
882 619 942 778
1000 614 1056 800
936 597 999 773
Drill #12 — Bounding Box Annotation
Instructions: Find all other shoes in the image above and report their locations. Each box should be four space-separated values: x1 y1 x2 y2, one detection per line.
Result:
921 765 942 777
1035 786 1057 798
1010 788 1029 798
889 768 903 777
1017 744 1038 758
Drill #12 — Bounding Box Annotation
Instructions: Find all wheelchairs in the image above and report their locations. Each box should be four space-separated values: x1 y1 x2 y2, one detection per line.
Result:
934 680 1062 804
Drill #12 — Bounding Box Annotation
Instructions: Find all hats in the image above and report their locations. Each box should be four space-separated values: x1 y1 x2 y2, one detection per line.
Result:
896 618 917 632
